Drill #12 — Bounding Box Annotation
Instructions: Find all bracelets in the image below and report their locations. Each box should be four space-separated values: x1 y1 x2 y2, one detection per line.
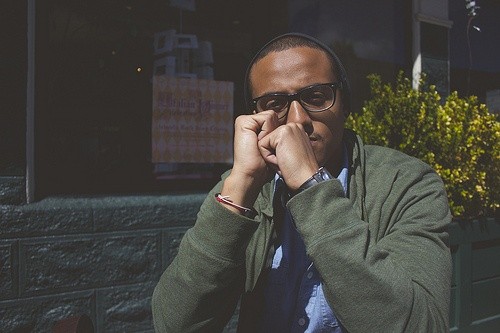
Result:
218 195 253 212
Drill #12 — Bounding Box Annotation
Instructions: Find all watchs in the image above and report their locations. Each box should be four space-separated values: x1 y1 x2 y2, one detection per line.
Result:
291 166 334 200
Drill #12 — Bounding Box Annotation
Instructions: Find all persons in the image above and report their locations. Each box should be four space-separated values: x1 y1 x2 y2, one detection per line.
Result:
150 32 454 332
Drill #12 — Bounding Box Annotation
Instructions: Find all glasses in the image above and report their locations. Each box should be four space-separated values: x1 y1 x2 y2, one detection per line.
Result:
252 81 342 120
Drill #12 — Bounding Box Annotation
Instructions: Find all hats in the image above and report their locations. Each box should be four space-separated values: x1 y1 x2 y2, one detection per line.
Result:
243 33 353 123
